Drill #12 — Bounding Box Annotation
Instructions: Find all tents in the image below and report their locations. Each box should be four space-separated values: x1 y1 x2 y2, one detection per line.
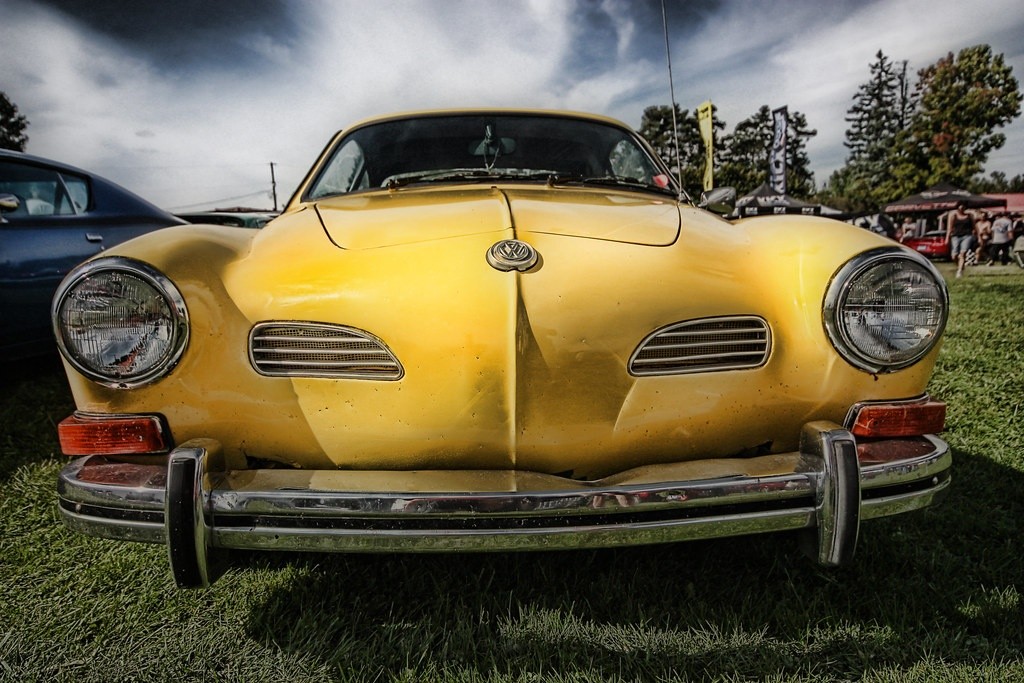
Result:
721 180 842 220
881 183 1007 227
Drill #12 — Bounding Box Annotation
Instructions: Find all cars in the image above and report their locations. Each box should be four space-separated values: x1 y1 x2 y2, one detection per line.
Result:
50 103 953 590
174 212 281 230
908 229 952 262
0 148 191 410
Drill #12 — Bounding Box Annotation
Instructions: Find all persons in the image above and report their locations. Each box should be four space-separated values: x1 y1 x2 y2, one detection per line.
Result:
899 216 917 243
972 211 992 266
944 201 983 279
986 211 1013 267
988 212 1024 268
